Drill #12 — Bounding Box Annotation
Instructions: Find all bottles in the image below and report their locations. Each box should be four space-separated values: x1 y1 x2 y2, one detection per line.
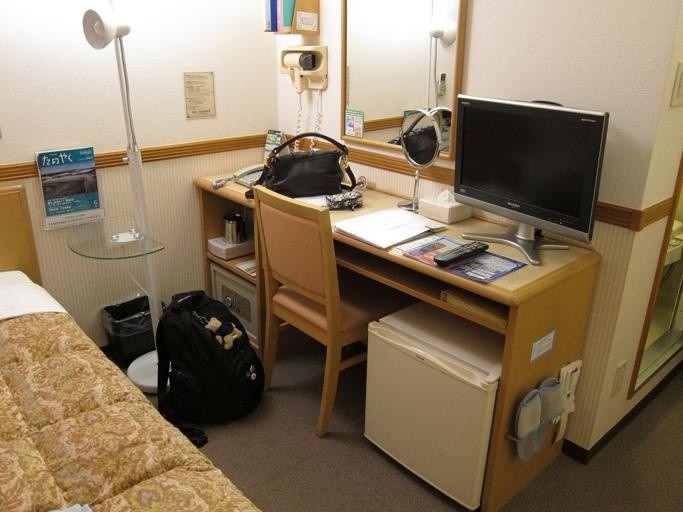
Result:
224 214 248 244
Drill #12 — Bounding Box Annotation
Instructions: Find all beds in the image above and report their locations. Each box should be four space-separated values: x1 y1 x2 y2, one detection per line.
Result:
1 183 263 512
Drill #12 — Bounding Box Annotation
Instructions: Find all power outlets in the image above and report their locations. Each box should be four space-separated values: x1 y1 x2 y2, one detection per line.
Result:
611 361 625 396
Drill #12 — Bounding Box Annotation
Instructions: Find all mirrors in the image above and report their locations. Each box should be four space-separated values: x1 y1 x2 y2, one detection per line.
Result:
339 0 469 211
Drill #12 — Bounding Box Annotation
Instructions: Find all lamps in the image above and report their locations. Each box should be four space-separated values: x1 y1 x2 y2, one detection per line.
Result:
427 22 456 111
67 9 172 395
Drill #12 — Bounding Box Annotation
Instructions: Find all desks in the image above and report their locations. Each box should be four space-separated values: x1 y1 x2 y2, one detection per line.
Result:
196 167 602 512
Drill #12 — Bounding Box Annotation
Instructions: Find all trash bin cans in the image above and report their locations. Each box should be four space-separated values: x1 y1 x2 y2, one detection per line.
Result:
102 294 168 370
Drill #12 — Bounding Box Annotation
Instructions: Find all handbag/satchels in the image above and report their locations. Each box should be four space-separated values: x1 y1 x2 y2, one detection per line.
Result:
324 192 362 211
246 133 356 201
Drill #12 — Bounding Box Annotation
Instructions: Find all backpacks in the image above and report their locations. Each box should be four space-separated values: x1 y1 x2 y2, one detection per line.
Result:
154 290 266 447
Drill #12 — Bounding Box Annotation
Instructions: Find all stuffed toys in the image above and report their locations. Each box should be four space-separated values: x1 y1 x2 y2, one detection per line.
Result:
203 316 242 350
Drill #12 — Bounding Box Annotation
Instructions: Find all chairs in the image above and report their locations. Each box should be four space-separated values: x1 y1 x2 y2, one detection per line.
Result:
248 184 413 438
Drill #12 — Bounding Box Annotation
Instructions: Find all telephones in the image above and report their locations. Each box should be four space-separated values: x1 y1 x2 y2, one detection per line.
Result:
235 163 265 188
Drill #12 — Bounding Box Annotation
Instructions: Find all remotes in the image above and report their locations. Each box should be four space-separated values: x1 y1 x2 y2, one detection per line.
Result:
433 240 490 264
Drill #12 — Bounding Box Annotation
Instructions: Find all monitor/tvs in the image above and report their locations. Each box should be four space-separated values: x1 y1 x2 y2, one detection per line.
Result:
452 92 610 263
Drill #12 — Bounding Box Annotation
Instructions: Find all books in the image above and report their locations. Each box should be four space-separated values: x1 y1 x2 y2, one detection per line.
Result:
438 286 509 327
333 207 447 251
34 146 101 228
261 0 296 34
